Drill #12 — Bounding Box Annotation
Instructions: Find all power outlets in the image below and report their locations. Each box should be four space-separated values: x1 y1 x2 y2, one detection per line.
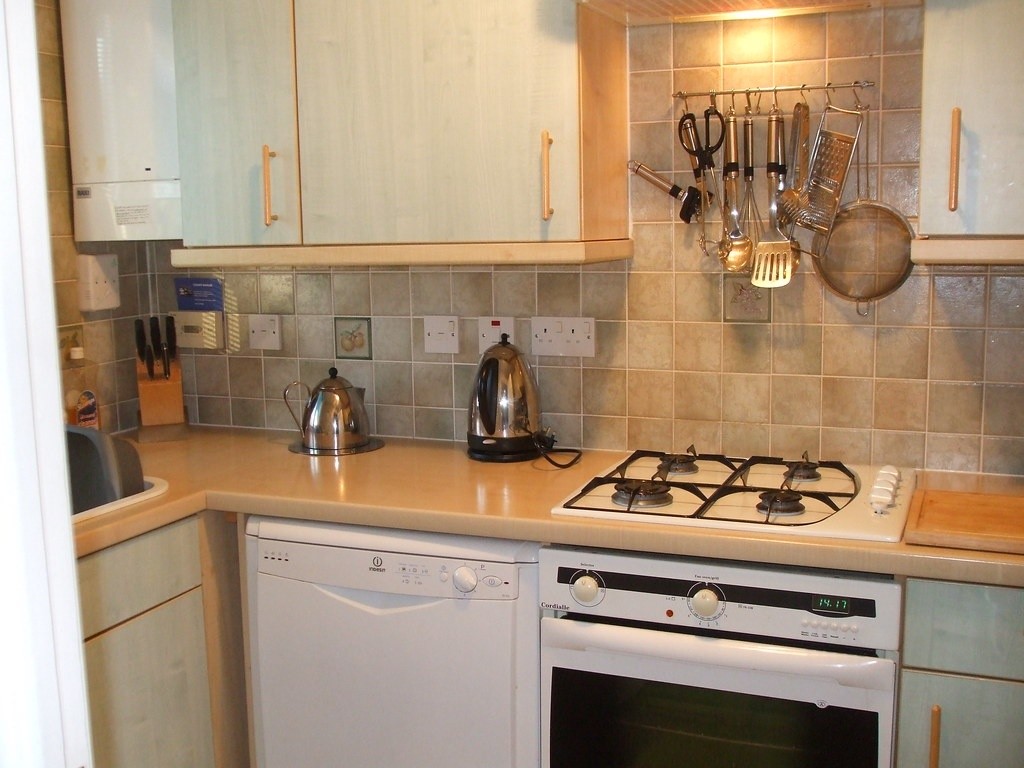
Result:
424 316 459 353
478 316 514 355
248 312 283 351
531 316 595 358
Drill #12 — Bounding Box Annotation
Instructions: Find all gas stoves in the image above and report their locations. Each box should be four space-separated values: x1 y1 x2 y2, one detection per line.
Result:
551 445 916 543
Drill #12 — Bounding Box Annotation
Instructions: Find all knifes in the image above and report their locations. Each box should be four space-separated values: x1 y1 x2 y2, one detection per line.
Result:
133 316 177 381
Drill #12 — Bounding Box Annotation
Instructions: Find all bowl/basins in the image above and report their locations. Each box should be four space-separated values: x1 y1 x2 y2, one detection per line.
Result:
67 426 144 514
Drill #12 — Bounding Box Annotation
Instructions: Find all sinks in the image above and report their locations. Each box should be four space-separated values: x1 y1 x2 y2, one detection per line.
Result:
72 476 172 525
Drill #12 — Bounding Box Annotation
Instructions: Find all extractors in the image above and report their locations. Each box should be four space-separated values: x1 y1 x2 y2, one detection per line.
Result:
577 0 924 37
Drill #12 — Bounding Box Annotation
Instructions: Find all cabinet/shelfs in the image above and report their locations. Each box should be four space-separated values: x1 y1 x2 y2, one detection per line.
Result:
170 0 632 268
910 0 1024 265
75 513 220 768
891 574 1024 768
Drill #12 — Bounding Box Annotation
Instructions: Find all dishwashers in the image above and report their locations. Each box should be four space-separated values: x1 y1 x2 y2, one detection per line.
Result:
241 516 542 768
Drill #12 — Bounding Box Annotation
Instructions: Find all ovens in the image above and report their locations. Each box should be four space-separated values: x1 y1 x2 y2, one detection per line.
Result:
537 547 899 768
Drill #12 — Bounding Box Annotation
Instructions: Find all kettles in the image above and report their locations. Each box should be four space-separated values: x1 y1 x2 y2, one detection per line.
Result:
465 331 549 464
283 368 370 450
